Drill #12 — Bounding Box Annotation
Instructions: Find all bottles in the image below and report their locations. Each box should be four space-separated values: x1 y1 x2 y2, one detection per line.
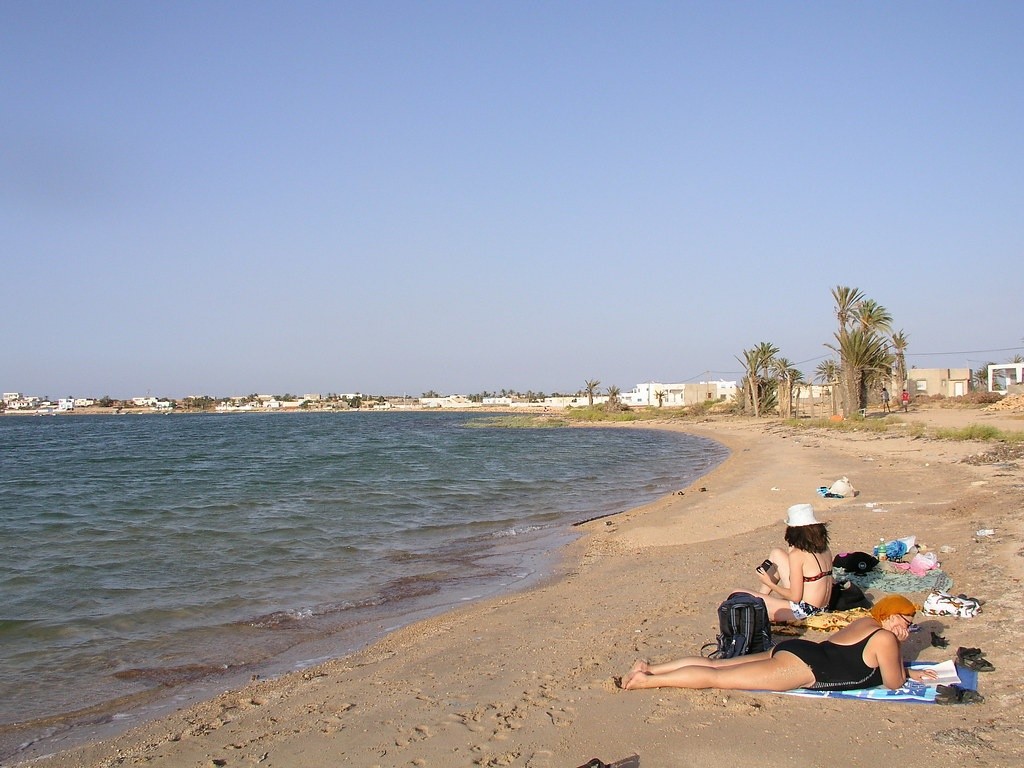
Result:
878 538 886 562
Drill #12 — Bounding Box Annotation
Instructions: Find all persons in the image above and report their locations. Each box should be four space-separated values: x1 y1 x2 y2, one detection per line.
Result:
881 387 891 412
733 504 833 621
621 594 939 692
900 389 909 412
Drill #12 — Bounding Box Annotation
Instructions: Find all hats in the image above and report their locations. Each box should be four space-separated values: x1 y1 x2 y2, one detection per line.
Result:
783 504 826 527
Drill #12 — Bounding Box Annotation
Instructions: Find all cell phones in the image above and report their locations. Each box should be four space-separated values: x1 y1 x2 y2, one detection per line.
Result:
756 559 772 574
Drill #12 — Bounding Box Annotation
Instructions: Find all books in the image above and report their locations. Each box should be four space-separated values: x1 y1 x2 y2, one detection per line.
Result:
922 660 961 685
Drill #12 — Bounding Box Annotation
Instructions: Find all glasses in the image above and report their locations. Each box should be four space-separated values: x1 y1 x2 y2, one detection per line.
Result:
898 613 913 628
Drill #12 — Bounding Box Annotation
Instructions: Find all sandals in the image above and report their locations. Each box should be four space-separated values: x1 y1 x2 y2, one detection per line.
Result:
954 646 995 672
936 681 985 705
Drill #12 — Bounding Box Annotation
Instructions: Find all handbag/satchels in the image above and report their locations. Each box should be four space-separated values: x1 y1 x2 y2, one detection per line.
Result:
921 589 981 619
828 579 871 612
833 552 879 577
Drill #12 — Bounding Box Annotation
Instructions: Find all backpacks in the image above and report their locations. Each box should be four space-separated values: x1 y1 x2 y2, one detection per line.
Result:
700 592 771 660
